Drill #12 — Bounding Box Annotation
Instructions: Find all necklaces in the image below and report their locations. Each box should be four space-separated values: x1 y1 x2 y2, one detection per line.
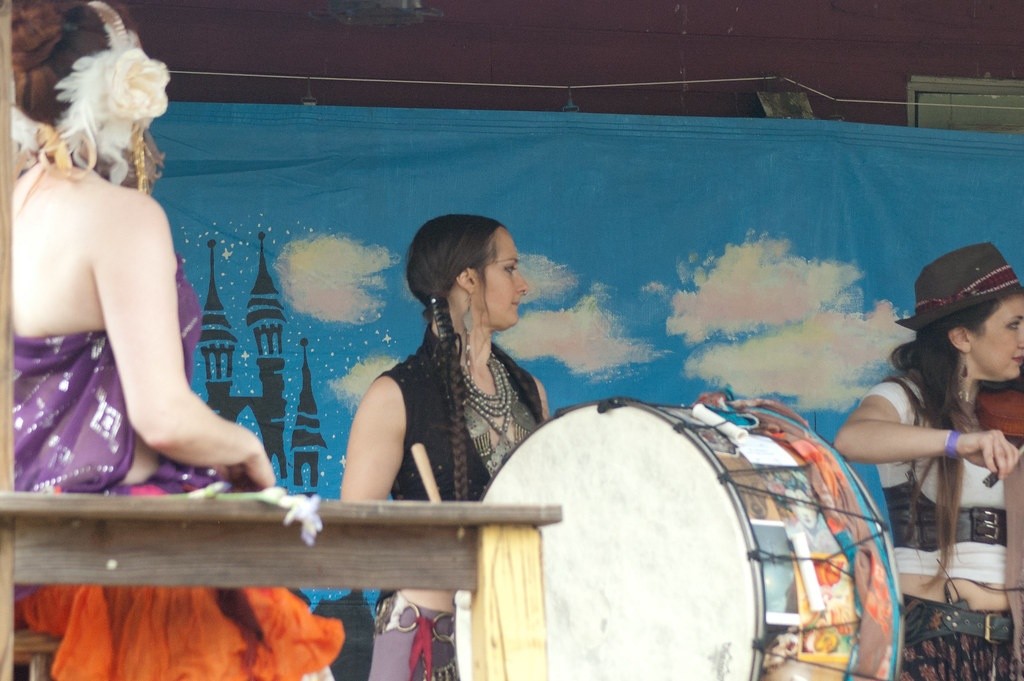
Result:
462 353 518 469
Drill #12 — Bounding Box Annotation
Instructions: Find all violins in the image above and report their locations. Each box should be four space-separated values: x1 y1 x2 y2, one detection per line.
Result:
973 374 1023 448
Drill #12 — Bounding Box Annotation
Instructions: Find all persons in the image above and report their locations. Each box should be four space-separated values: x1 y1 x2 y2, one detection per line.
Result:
11 0 346 681
340 213 550 681
834 242 1024 681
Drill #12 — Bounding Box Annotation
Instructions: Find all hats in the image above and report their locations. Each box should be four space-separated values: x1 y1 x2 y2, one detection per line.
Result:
895 243 1024 331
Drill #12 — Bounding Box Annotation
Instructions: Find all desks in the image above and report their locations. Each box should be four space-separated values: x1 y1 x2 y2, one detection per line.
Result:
1 486 562 681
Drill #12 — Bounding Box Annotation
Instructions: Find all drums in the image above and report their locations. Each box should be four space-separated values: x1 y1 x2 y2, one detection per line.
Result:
454 394 908 681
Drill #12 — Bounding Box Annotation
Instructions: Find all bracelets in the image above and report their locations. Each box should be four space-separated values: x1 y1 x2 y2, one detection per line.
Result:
944 429 963 460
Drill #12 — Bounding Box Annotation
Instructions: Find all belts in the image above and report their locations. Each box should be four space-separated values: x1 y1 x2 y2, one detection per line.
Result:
902 598 1015 647
